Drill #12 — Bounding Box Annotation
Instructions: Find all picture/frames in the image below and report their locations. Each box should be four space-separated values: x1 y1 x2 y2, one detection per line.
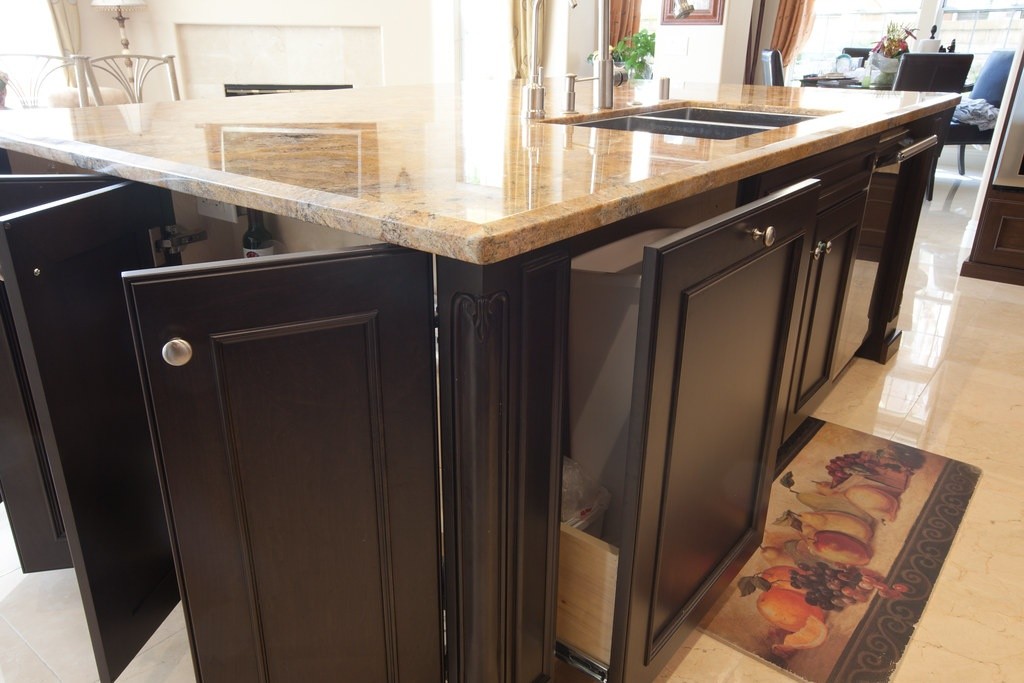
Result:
660 0 725 25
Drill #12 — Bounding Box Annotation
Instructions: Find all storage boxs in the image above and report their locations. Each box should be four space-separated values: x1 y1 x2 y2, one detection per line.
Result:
567 225 684 546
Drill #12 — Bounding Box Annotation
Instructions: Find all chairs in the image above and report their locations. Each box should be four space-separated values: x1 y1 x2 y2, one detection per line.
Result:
762 48 785 86
892 53 974 201
0 52 181 109
944 49 1015 175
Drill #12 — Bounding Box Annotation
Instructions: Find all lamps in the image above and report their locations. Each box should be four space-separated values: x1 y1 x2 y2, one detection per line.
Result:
91 0 146 87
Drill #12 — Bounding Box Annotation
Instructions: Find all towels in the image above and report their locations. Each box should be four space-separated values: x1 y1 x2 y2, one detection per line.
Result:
951 98 1000 131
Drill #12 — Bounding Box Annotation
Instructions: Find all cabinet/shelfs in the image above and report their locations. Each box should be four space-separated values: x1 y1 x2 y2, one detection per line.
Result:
0 109 956 683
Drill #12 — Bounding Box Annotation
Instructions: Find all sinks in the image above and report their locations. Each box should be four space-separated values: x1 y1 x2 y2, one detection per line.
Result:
563 115 781 141
631 106 827 129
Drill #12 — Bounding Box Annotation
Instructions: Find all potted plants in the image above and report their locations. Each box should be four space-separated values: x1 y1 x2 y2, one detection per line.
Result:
586 30 656 79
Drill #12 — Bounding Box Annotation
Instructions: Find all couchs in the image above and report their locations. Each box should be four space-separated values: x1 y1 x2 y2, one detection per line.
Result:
40 85 127 109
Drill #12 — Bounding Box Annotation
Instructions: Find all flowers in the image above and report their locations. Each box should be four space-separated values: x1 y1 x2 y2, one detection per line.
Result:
868 20 920 74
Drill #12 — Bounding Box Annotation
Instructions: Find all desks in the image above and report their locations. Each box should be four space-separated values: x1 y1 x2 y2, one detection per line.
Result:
801 76 975 94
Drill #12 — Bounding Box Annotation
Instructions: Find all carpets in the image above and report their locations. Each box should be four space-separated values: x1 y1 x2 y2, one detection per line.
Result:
693 415 983 683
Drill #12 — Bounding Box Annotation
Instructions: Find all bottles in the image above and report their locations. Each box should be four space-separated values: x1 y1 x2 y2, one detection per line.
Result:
243 208 274 258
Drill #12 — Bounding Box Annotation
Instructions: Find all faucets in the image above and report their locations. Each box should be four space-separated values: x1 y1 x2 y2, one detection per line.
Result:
591 0 630 110
520 0 579 119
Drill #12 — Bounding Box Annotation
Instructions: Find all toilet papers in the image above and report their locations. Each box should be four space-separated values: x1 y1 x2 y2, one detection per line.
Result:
915 39 941 53
